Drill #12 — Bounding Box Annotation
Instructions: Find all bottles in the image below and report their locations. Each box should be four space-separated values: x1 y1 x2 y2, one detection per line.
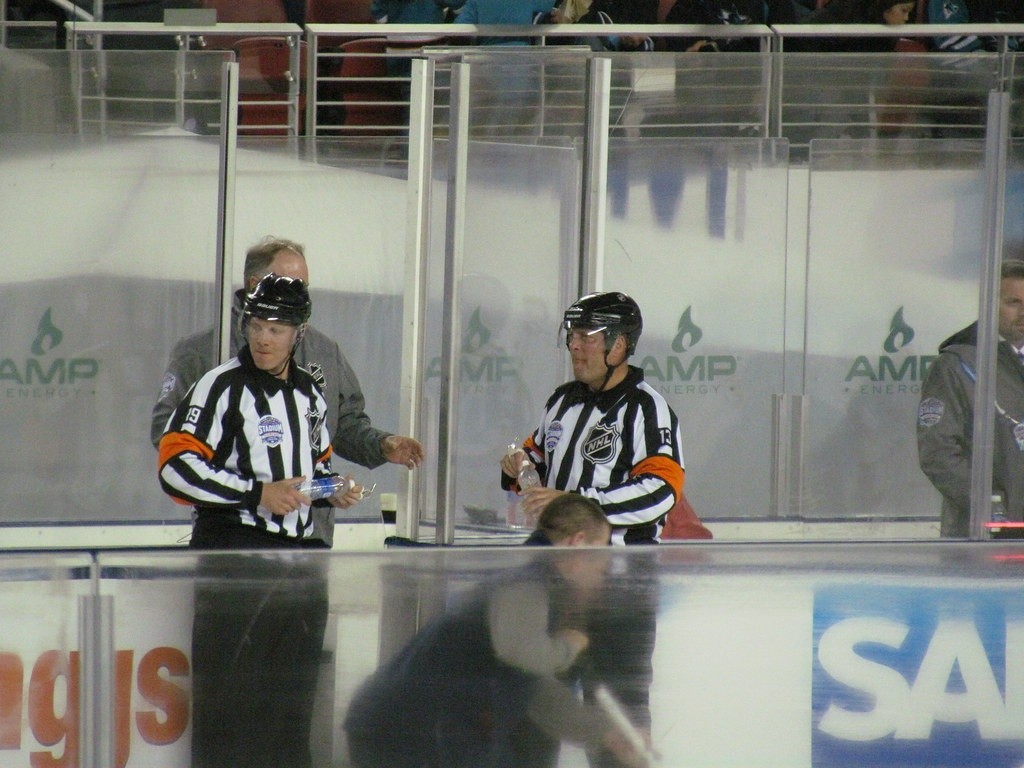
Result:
517 460 542 496
990 494 1008 539
296 475 356 499
508 491 528 529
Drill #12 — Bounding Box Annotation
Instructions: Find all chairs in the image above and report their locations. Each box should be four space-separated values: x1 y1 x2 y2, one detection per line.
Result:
200 0 307 136
303 0 407 134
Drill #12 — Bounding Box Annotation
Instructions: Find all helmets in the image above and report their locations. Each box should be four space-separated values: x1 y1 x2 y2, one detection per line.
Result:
563 291 643 355
241 272 312 330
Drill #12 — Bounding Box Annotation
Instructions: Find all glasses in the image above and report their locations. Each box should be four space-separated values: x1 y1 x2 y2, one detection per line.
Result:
566 332 606 344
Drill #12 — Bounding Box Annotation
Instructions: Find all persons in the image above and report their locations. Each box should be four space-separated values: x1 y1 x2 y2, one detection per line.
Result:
342 492 664 767
148 233 427 768
499 290 686 768
917 257 1024 539
371 1 1024 151
